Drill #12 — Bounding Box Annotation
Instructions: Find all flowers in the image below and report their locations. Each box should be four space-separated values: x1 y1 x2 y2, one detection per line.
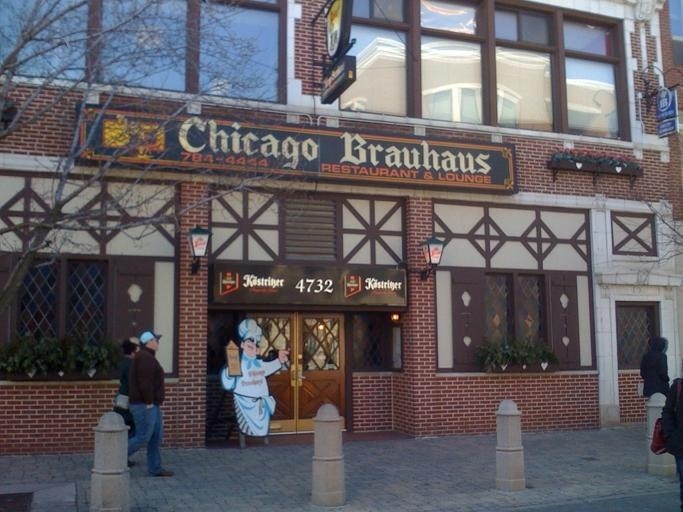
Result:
552 147 599 165
597 150 640 168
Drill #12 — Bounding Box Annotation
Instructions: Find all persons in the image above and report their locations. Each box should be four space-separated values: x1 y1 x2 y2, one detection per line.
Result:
114 336 140 466
639 336 670 397
128 328 176 476
660 377 683 512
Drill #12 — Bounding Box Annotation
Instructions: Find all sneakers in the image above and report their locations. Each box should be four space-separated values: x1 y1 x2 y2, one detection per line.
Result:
149 469 174 476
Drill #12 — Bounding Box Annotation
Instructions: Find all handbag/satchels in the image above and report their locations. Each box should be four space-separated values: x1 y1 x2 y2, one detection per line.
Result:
114 407 136 438
650 418 667 455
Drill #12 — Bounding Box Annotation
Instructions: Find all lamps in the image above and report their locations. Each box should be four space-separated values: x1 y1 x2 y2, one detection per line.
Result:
419 236 446 280
187 224 211 272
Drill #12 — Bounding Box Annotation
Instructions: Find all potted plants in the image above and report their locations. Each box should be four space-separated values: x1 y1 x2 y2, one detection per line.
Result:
0 336 118 381
476 334 560 373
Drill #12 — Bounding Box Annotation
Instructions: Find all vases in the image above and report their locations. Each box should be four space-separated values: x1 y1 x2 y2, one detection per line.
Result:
597 164 644 189
549 160 596 187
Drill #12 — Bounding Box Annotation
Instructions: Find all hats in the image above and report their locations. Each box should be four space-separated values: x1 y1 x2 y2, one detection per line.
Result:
121 330 162 345
